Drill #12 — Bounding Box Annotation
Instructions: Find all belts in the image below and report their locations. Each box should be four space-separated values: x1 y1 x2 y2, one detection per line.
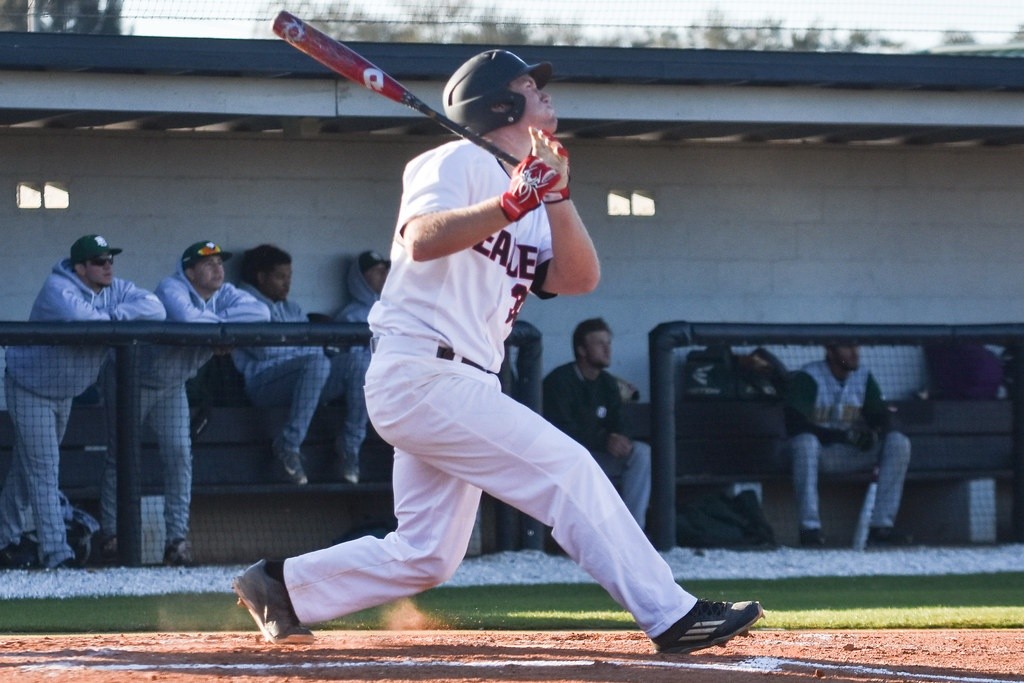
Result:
436 348 492 371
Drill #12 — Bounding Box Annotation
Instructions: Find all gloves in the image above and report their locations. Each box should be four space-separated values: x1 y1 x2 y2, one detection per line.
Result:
528 127 570 204
499 156 560 221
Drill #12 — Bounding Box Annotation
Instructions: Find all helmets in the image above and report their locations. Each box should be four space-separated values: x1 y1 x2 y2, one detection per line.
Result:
443 49 552 139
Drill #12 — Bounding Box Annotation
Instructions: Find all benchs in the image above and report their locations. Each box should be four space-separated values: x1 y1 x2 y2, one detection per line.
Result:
0 400 1023 566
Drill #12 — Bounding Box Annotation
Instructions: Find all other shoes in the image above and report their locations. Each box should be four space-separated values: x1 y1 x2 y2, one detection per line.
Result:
868 527 914 547
163 541 193 566
102 537 118 561
803 532 828 547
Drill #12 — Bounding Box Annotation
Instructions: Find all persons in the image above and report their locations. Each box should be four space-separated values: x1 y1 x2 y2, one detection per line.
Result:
233 50 763 655
0 233 166 569
230 244 369 485
103 240 271 568
321 250 392 352
686 342 789 548
785 343 913 546
543 316 651 532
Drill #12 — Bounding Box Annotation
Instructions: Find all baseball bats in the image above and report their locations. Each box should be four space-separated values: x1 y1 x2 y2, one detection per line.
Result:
273 10 519 166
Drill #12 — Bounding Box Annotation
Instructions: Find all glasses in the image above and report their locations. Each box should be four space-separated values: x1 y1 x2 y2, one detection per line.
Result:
86 255 114 265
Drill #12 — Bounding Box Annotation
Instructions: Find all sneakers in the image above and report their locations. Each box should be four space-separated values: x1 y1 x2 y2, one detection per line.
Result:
653 598 763 655
336 466 361 483
275 445 308 485
234 559 314 644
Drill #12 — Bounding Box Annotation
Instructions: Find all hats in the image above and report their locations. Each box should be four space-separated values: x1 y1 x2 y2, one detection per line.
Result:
71 234 123 263
181 241 232 268
360 251 391 271
